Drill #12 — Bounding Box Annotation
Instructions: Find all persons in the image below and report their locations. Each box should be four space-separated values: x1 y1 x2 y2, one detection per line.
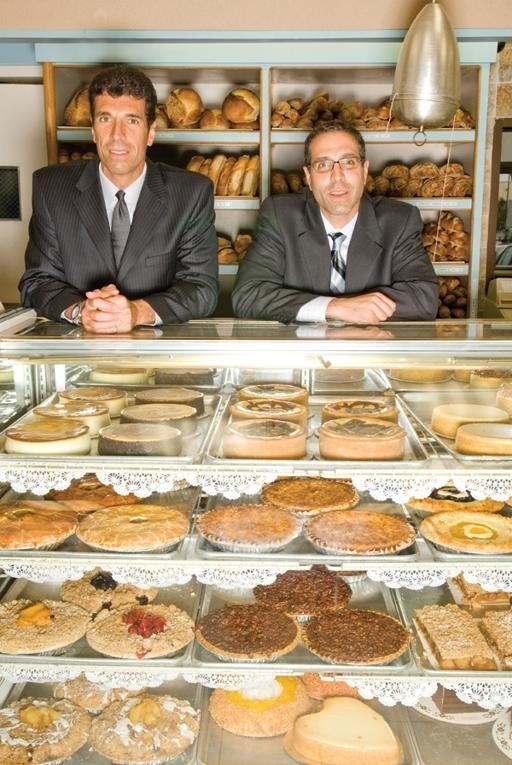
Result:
230 124 440 324
19 65 219 334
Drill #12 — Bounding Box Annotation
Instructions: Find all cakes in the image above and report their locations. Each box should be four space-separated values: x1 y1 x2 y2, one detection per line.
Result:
98 422 182 457
134 388 206 417
389 369 451 381
314 369 366 383
222 419 308 460
253 570 353 622
404 480 506 520
42 672 147 715
90 368 148 385
453 367 498 381
238 370 295 385
302 509 416 555
194 600 302 663
303 672 359 700
470 368 512 388
293 697 402 764
419 509 512 555
90 694 199 763
195 503 304 553
0 500 78 551
118 404 197 436
76 503 190 552
258 476 361 520
478 607 512 668
57 386 128 417
495 382 512 417
60 567 159 615
320 401 400 432
239 383 309 413
311 564 368 585
505 495 512 516
319 417 407 460
430 403 510 438
453 572 511 607
33 402 112 436
153 369 213 385
415 604 500 670
301 607 413 666
0 599 92 656
431 684 490 714
229 398 308 433
455 423 512 455
5 419 90 456
0 695 89 765
210 675 311 736
86 602 195 660
43 474 143 518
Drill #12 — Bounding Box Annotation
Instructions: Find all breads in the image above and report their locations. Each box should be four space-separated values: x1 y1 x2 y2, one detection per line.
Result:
374 176 390 196
233 235 253 260
382 165 410 180
401 179 423 198
240 155 261 197
271 171 289 194
423 233 434 248
154 103 171 130
215 235 237 265
437 276 467 318
82 152 97 161
389 177 408 197
200 109 231 129
438 212 464 234
439 163 467 178
207 154 227 196
228 154 250 197
410 161 442 180
421 177 455 198
449 233 471 248
364 175 376 196
271 91 418 128
446 255 464 263
303 176 310 187
231 121 260 131
445 242 466 260
424 222 449 244
172 123 201 129
197 158 213 177
185 156 205 173
166 87 204 126
285 172 303 193
417 178 444 198
58 150 68 164
222 88 260 123
63 82 92 127
431 253 448 262
70 153 81 162
449 175 473 198
427 242 448 256
447 106 475 129
216 156 237 196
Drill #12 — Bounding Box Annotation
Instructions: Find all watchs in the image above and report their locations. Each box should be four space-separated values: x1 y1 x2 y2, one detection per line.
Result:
70 299 87 324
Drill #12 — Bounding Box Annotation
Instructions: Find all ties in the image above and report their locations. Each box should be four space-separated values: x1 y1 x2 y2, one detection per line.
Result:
110 190 131 271
327 232 347 296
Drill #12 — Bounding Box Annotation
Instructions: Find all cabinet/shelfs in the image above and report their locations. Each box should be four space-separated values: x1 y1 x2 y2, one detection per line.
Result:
34 42 497 323
2 307 512 709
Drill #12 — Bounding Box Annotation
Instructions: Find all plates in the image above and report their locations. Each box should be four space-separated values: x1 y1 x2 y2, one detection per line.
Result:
0 564 205 668
0 670 197 762
404 485 511 564
493 707 512 759
394 572 435 672
411 698 505 724
1 377 50 433
192 668 411 764
396 391 512 469
206 382 432 472
2 474 206 560
194 478 422 562
2 384 224 465
190 569 415 676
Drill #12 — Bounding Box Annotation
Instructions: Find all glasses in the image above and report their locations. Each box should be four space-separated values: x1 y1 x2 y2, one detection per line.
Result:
307 157 363 173
